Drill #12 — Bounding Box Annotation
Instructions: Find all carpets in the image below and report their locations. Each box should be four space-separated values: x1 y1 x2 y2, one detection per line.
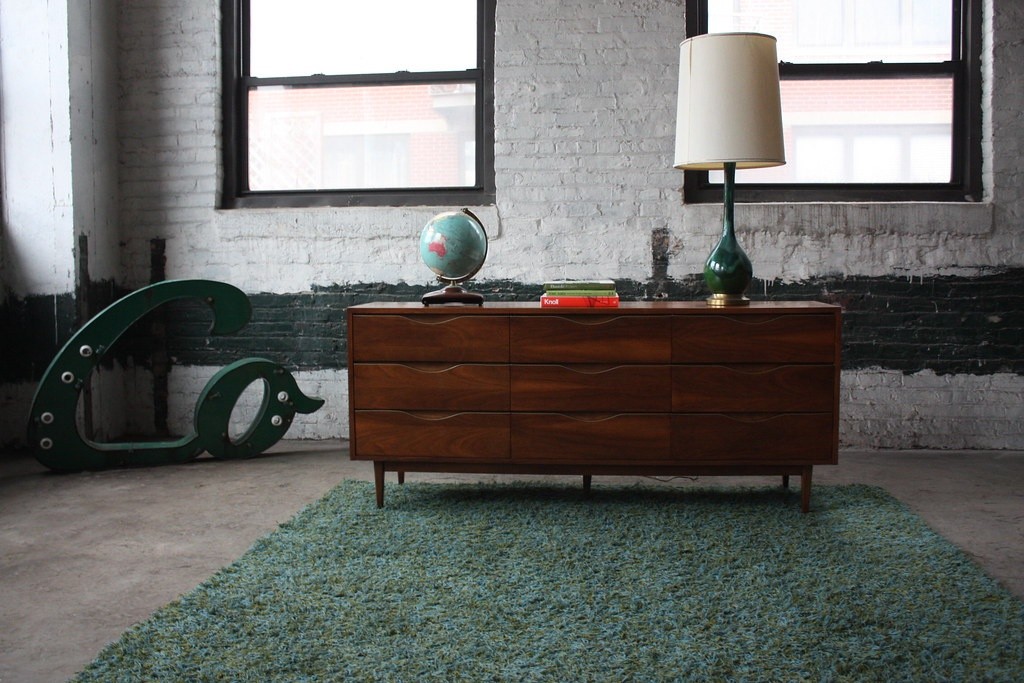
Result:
67 477 1024 683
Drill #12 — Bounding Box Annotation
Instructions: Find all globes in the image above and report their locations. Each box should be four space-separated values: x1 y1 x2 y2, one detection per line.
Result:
418 207 488 307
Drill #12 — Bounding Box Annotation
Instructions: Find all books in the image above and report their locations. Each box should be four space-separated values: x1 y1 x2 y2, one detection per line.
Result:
539 279 619 308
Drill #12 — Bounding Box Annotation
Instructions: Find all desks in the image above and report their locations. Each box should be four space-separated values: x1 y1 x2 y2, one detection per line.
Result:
347 300 842 512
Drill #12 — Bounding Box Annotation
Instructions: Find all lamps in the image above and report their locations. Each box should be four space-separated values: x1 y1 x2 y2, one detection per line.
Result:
672 31 785 306
